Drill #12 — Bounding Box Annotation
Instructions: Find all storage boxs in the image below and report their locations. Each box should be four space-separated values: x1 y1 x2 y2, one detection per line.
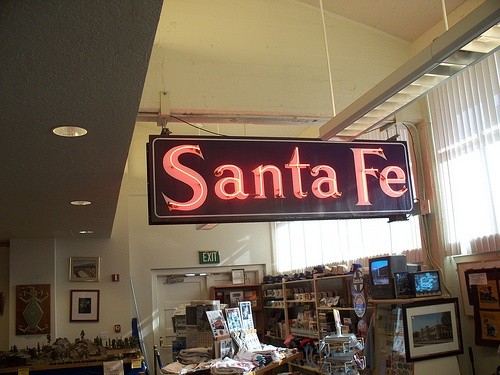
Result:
172 300 220 358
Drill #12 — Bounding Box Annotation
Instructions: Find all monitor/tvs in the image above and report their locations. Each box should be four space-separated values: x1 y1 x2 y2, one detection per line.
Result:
369 255 407 298
413 271 442 297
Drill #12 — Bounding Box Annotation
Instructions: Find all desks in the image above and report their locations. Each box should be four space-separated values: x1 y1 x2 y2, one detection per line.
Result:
0 356 144 375
248 350 304 375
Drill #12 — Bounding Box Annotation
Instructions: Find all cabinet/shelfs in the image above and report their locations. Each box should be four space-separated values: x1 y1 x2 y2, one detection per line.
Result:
261 273 368 375
210 284 263 343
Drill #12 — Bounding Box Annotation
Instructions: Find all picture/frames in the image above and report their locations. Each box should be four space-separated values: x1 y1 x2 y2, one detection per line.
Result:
69 256 101 281
70 289 99 322
401 297 465 363
